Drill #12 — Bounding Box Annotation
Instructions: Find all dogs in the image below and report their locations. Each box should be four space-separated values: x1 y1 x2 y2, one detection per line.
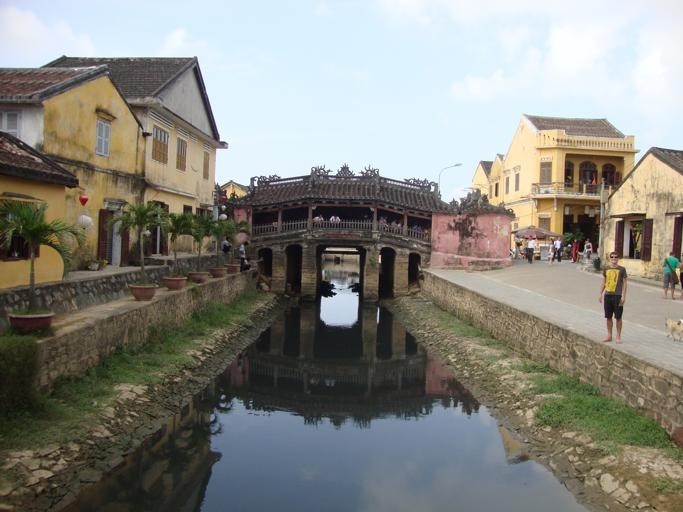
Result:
665 317 683 342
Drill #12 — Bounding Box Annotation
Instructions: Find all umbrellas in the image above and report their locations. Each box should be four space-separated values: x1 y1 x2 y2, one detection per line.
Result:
511 225 558 241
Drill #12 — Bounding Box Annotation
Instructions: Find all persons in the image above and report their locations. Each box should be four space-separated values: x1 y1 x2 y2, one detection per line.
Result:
377 216 427 239
221 237 233 264
328 214 340 227
510 233 592 264
311 212 323 227
598 252 626 343
238 240 249 271
660 252 681 299
679 253 683 300
240 257 251 271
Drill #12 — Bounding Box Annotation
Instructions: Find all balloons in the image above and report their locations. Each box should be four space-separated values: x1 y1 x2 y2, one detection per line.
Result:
79 194 88 206
77 215 91 230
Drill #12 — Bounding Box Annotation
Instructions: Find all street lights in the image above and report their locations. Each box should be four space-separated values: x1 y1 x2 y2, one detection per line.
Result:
438 161 465 194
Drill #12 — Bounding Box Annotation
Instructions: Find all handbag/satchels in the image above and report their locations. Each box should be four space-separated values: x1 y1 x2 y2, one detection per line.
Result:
671 271 680 284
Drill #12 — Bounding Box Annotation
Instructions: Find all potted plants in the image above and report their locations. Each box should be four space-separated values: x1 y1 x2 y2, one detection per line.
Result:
163 208 245 289
104 201 167 300
0 200 86 335
77 257 105 271
